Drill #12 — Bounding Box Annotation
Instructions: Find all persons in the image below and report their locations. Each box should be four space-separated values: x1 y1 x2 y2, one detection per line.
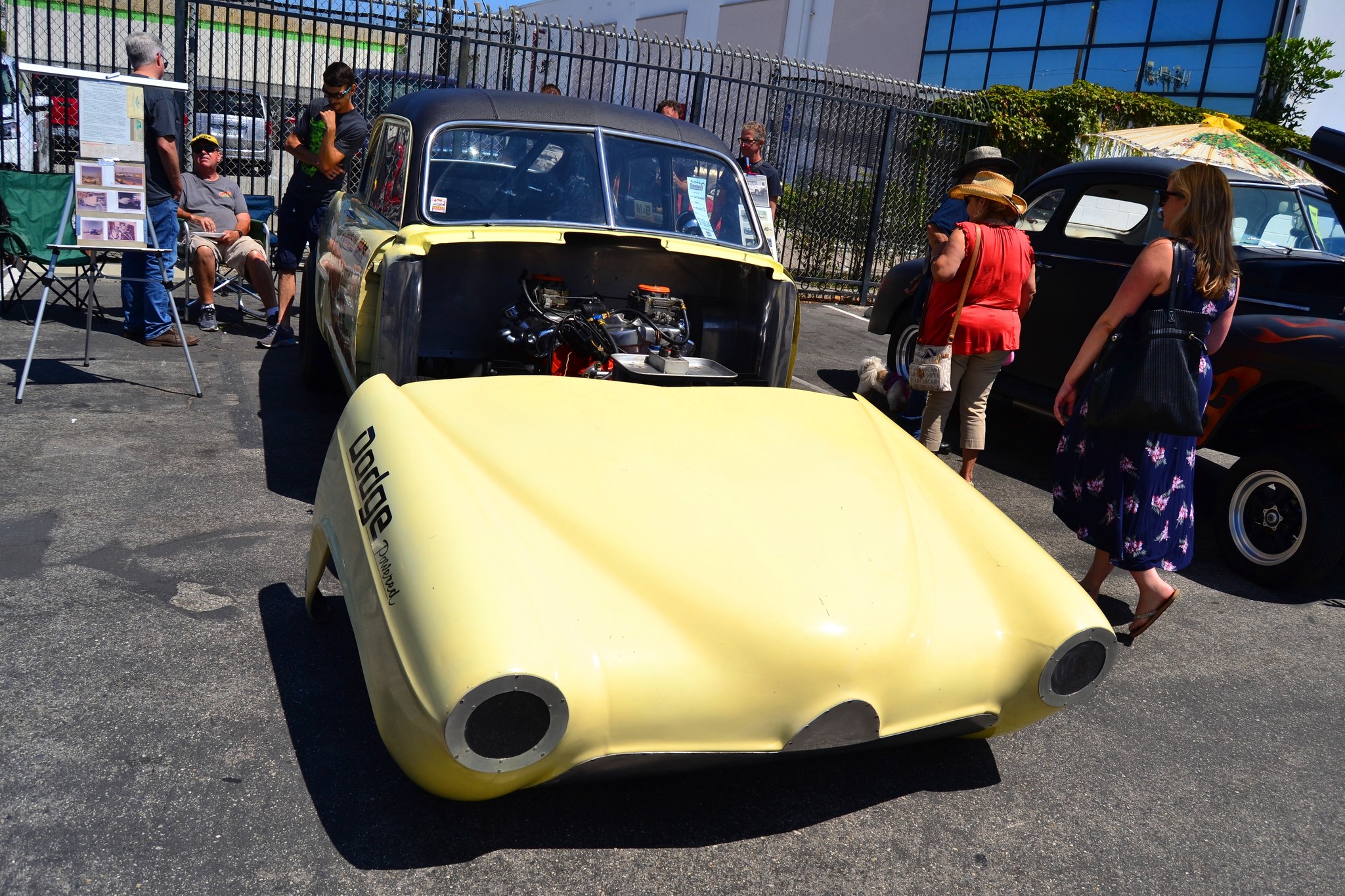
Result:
1054 164 1241 639
900 146 1020 455
918 171 1036 487
614 100 695 215
258 62 367 349
124 32 200 348
1282 126 1345 254
176 135 294 333
709 122 783 247
540 84 561 95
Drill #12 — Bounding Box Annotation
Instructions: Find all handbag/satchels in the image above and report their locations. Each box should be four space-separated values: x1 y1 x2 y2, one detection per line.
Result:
1085 236 1210 437
909 343 952 392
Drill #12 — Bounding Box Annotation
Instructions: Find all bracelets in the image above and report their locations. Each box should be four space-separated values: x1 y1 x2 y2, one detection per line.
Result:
233 230 243 237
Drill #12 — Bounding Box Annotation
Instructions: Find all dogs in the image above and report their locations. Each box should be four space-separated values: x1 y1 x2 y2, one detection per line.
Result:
855 355 912 415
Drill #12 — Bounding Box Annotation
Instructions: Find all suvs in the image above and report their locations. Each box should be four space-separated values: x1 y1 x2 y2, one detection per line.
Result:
194 84 277 176
1 53 80 172
343 68 486 200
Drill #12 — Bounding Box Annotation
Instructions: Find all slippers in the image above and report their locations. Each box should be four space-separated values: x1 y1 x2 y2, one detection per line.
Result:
1129 589 1181 638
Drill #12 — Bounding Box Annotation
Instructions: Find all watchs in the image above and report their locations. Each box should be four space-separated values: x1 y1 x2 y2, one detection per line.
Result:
930 255 940 265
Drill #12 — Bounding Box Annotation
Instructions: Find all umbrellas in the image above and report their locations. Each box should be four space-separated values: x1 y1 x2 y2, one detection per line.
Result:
1081 112 1334 194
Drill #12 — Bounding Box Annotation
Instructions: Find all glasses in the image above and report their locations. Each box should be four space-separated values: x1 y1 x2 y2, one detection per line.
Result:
982 167 1006 177
1158 189 1185 205
157 53 169 69
964 195 979 206
192 145 219 153
738 138 758 145
322 83 351 99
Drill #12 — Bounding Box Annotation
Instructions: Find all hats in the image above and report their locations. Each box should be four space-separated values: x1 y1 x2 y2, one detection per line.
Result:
948 171 1028 228
956 146 1021 177
189 134 219 148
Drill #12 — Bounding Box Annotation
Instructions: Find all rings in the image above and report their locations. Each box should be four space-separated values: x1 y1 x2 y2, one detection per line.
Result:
331 173 334 176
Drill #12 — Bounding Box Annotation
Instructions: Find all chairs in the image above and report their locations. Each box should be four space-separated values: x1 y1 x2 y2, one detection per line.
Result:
1230 208 1345 256
0 168 286 327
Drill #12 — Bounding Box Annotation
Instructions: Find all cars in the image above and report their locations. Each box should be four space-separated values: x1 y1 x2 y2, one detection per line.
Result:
866 156 1345 601
269 101 310 151
297 88 775 398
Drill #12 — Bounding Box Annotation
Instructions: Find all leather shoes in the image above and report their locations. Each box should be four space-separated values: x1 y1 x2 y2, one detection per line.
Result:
122 327 143 338
143 325 200 346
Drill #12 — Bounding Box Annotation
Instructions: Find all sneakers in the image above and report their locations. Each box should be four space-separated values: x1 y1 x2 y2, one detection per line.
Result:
199 304 219 331
266 311 294 335
257 323 296 349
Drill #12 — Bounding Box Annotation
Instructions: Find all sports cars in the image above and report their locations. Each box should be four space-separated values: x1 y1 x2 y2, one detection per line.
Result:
304 217 1139 809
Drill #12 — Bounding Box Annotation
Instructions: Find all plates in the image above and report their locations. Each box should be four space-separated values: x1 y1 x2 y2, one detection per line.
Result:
190 232 227 238
